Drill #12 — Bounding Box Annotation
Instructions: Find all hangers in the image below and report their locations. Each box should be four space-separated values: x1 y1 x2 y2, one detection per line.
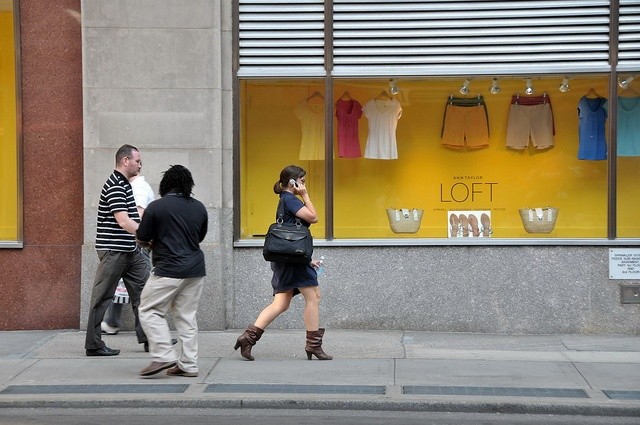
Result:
375 90 392 101
340 90 354 105
618 85 639 97
295 91 325 107
585 88 601 101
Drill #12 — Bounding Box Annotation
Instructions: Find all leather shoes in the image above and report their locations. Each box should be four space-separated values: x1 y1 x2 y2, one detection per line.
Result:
166 365 198 377
86 346 120 356
144 339 179 352
138 361 177 376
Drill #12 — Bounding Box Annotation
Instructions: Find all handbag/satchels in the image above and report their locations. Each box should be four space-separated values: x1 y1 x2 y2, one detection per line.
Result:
263 193 313 265
386 208 424 233
519 208 559 233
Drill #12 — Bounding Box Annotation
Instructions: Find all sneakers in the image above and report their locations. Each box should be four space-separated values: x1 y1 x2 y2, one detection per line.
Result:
100 321 120 335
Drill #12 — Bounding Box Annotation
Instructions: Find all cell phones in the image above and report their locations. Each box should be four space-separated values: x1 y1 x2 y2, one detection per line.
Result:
289 179 298 188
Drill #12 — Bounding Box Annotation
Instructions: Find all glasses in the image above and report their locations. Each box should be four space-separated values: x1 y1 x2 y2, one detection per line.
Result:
301 179 305 182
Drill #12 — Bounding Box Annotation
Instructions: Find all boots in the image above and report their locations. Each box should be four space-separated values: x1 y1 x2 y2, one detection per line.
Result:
234 323 265 361
305 328 333 360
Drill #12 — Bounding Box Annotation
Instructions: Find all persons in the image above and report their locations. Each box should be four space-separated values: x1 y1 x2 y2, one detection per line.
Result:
234 165 332 360
84 144 177 356
101 158 155 335
135 165 208 377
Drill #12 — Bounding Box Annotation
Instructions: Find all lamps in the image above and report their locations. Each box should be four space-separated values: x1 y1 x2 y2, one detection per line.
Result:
488 78 500 95
522 76 536 94
458 76 473 95
618 75 635 90
387 77 400 96
558 77 572 93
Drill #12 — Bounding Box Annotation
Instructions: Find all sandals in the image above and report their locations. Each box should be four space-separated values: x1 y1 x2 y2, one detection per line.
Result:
459 214 469 237
449 214 460 238
468 214 481 237
481 213 493 237
